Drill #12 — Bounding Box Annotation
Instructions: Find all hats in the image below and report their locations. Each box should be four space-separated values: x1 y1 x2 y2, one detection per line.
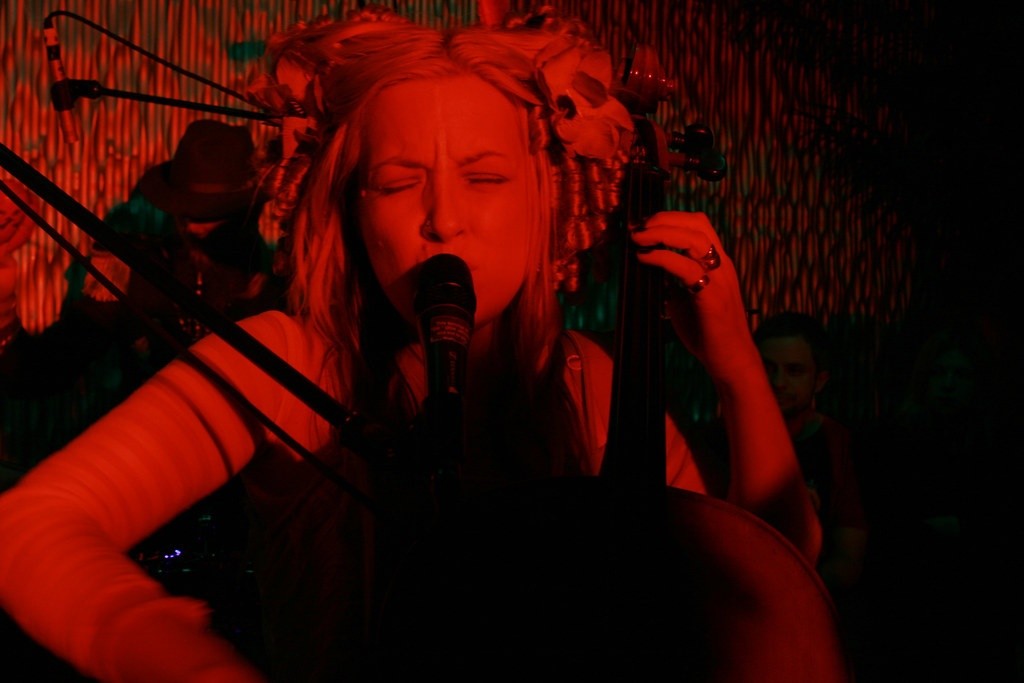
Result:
139 118 260 222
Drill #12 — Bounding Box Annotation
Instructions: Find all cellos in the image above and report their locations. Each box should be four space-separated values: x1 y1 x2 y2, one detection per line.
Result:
214 42 852 683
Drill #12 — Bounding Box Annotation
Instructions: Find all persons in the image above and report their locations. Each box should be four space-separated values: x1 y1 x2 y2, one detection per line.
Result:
1 21 821 683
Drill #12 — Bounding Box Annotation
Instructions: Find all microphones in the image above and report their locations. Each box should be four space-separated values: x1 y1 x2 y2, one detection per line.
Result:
43 16 77 147
412 254 476 416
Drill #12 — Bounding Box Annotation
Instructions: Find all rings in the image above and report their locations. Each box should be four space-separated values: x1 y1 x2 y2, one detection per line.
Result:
700 244 721 271
685 272 711 296
